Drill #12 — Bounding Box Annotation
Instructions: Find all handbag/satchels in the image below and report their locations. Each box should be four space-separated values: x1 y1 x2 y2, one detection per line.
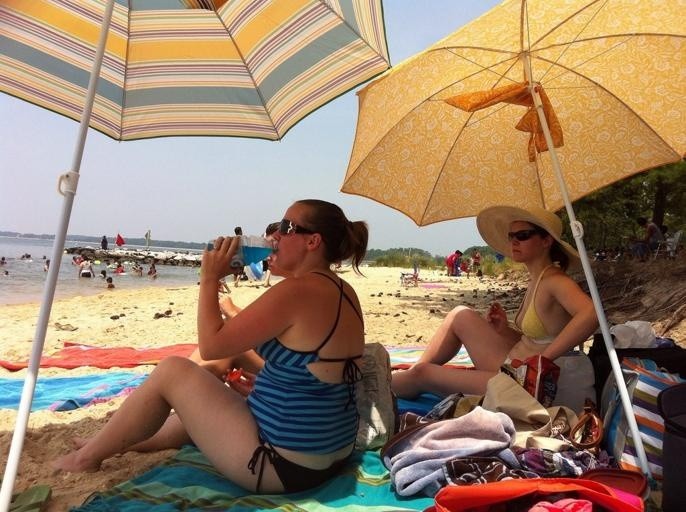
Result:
598 353 686 482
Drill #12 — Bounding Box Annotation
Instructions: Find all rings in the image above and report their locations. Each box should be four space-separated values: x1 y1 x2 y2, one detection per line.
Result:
206 242 217 252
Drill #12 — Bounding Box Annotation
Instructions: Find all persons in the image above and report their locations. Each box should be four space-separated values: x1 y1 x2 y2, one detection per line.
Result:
0 252 50 277
144 228 152 250
657 224 667 243
99 236 109 250
390 219 601 402
103 220 296 419
217 277 233 294
41 197 373 499
444 246 483 278
633 216 670 262
260 232 273 289
71 254 158 290
232 225 244 288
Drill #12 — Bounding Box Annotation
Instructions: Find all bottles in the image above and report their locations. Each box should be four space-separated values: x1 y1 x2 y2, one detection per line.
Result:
207 236 278 269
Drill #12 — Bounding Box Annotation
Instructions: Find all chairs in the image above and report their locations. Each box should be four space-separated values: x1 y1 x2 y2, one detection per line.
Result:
652 239 676 260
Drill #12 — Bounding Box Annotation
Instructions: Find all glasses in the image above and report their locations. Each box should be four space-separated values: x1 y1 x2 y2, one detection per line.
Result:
509 229 541 242
277 218 314 237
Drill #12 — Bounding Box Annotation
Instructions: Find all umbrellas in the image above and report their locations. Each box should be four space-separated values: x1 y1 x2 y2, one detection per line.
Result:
0 0 393 509
337 0 686 489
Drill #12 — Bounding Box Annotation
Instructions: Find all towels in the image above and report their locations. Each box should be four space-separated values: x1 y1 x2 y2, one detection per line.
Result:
383 408 541 496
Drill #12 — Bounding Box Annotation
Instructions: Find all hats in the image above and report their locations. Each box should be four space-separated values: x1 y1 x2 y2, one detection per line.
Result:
475 203 602 271
